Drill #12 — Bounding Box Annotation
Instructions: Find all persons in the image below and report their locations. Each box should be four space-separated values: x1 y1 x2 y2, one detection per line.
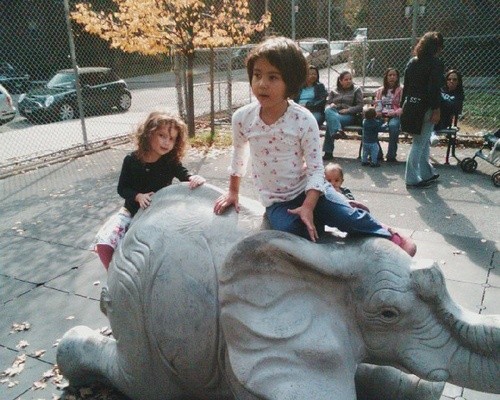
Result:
213 34 416 255
324 164 355 202
361 108 388 167
294 66 325 127
399 31 443 188
430 69 464 146
323 71 364 161
369 68 404 162
91 111 206 273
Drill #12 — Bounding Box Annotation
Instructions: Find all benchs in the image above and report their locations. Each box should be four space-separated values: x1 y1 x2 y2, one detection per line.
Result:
319 85 460 165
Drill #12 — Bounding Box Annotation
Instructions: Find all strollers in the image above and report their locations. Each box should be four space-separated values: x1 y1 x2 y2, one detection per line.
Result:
461 126 500 186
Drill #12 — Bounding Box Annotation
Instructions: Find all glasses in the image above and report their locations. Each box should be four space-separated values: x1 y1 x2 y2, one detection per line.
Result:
446 78 457 81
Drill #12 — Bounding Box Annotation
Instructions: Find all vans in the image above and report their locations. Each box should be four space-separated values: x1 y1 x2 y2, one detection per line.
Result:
296 39 330 69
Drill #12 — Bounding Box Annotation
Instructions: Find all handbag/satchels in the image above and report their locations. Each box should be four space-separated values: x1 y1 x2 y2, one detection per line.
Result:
399 96 430 136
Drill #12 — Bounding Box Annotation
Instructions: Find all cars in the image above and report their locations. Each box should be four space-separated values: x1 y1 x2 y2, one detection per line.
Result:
329 41 352 66
0 84 16 126
215 44 257 70
18 66 132 123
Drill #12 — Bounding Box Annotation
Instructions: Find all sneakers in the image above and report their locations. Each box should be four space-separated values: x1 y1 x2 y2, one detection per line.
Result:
323 152 334 160
349 202 369 212
423 173 440 184
369 161 381 168
361 162 370 166
388 228 416 257
406 179 439 190
334 129 347 139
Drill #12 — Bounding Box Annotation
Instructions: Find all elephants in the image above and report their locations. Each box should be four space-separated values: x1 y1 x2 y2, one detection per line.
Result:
56 180 500 399
